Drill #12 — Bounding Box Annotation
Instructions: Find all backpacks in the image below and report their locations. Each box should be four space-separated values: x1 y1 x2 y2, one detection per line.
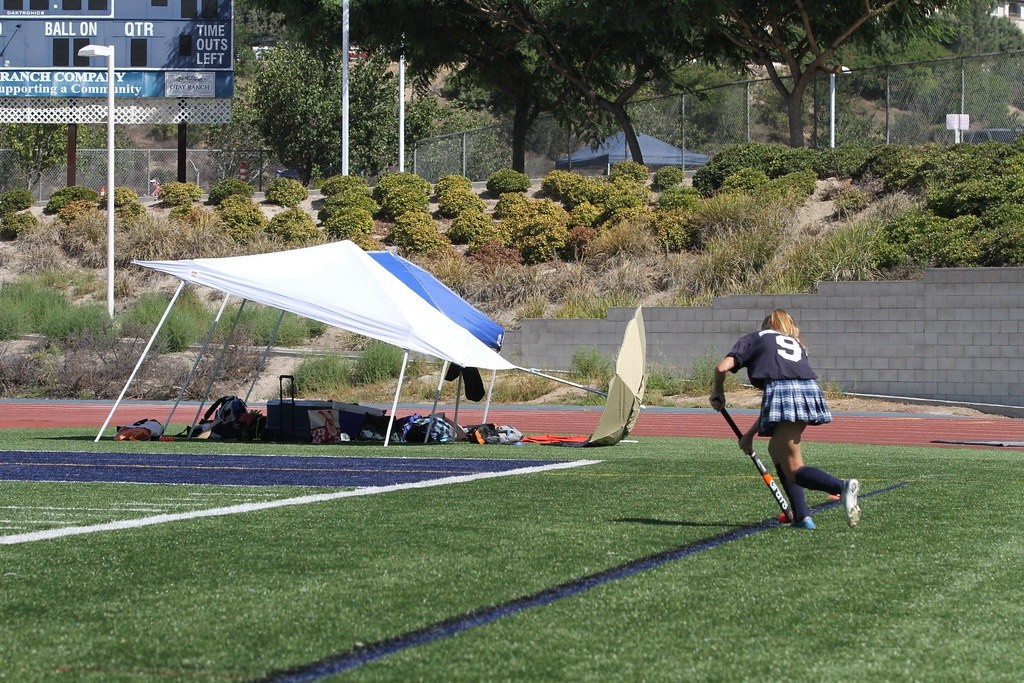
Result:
201 394 250 439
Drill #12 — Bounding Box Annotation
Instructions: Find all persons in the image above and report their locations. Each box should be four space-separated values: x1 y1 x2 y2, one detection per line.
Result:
149 178 160 196
710 310 860 530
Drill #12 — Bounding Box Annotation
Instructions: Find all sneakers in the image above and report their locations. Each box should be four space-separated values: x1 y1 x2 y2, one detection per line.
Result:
790 518 816 529
841 479 861 528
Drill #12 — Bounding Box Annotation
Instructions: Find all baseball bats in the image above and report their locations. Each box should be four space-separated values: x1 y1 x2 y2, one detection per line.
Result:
712 396 796 523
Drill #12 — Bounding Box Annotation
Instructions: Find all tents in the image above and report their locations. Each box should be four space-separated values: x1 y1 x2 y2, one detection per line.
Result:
189 251 504 443
555 129 709 175
95 239 516 447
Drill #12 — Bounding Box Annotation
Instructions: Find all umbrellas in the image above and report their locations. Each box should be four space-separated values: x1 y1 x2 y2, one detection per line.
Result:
515 306 646 445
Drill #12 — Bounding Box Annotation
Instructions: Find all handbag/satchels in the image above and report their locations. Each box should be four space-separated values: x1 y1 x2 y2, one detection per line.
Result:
308 410 341 444
116 418 164 441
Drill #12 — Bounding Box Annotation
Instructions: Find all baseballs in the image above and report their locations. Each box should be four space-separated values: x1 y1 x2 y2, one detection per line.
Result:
778 513 788 523
